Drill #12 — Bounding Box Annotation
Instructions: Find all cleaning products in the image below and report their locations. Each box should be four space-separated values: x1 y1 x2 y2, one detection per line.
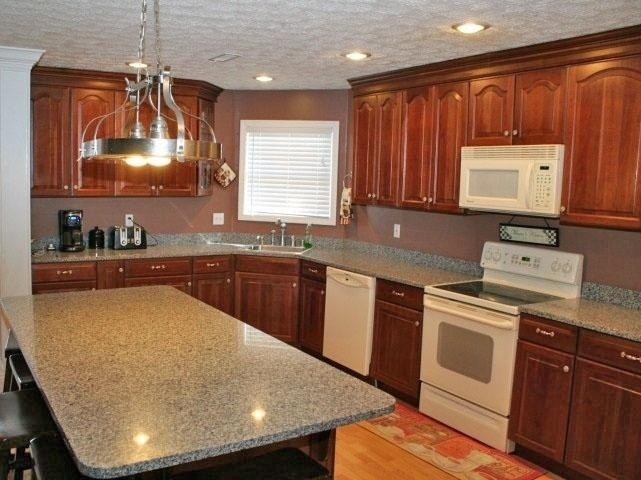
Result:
303 223 313 248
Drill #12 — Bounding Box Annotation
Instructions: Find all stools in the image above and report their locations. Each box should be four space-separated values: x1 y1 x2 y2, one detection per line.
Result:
28 430 83 480
1 390 64 480
9 355 36 390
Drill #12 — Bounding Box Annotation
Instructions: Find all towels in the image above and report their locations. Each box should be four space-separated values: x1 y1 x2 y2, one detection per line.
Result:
339 187 355 226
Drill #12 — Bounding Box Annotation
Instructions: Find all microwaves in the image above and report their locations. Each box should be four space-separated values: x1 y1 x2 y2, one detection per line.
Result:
459 144 565 218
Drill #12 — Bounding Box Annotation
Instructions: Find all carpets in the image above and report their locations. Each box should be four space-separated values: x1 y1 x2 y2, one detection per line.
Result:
357 400 547 480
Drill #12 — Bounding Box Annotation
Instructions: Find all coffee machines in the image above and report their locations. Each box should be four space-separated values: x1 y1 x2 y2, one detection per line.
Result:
60 209 83 253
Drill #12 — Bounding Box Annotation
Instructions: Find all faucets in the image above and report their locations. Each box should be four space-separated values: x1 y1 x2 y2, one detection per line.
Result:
270 217 297 247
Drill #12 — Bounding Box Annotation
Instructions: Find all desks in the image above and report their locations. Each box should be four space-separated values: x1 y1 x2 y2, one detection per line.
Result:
0 285 396 476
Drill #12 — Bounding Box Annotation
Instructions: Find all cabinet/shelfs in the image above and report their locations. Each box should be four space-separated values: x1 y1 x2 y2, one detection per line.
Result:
124 259 193 295
235 255 298 348
114 89 196 198
192 258 234 318
370 279 423 400
198 98 215 199
398 76 469 211
564 324 641 476
507 313 579 466
471 67 563 212
297 259 326 354
97 261 124 288
31 263 97 292
30 84 114 198
559 42 641 231
352 90 398 206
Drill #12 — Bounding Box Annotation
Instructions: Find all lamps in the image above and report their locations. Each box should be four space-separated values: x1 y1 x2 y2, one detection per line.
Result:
451 21 491 34
126 61 149 67
341 50 373 61
76 0 222 168
253 75 274 81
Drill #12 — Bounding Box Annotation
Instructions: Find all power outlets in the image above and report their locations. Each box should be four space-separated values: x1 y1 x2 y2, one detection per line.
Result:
213 213 225 225
125 214 133 227
394 223 400 238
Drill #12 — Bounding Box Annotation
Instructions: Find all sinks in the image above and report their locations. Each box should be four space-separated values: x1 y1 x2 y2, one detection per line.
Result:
246 244 312 254
